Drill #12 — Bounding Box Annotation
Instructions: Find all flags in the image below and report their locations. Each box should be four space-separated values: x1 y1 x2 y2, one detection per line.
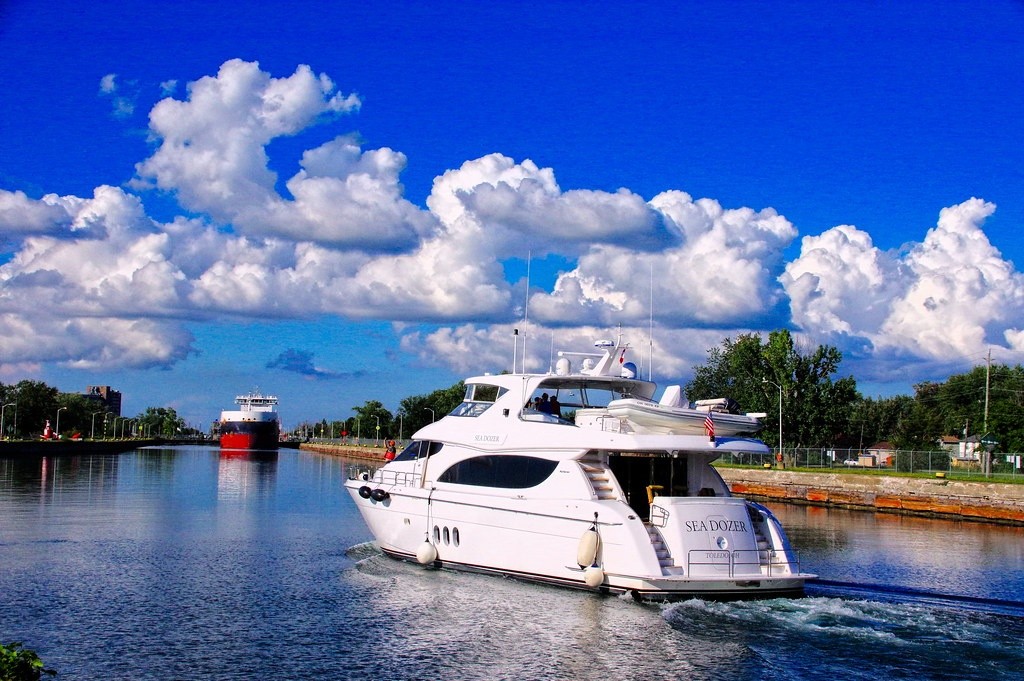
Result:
704 409 715 437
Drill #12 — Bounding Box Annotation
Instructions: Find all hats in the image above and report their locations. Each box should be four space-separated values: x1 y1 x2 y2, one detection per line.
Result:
388 441 394 445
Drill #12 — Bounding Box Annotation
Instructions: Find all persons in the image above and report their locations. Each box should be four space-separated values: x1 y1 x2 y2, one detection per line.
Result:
384 437 396 464
524 393 562 419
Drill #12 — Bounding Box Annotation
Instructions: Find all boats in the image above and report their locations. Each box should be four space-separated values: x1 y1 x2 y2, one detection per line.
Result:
341 248 822 602
218 386 282 450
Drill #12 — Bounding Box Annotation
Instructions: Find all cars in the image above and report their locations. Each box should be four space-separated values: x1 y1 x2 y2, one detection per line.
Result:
844 457 858 465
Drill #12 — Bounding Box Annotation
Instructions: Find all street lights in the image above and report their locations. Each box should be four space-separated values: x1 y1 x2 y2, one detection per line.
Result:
56 406 68 435
399 413 403 448
91 411 101 438
103 412 113 439
113 417 158 439
761 375 784 461
424 407 435 423
0 403 16 441
370 414 380 446
